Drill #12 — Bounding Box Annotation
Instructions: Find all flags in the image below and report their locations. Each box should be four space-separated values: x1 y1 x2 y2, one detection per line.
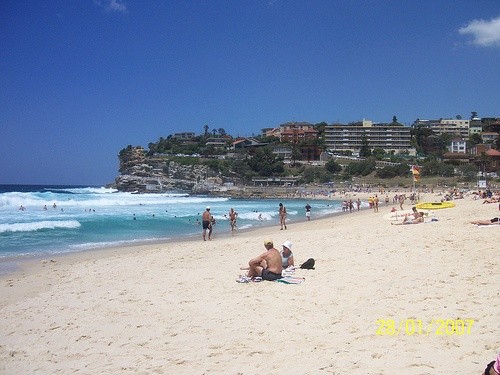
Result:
412 165 420 182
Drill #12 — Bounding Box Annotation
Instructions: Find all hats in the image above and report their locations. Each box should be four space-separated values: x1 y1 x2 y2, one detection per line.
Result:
206 207 210 210
264 239 273 245
281 241 292 252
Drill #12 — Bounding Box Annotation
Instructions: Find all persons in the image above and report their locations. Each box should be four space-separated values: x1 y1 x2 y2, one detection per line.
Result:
241 242 294 270
340 198 361 213
304 202 311 221
244 180 372 198
85 204 167 220
19 205 25 211
471 218 500 225
464 182 500 211
202 206 213 241
52 203 63 212
279 203 287 230
43 205 47 210
378 183 434 212
247 239 283 281
367 195 379 213
174 210 262 225
436 185 464 202
229 207 238 232
391 206 424 225
